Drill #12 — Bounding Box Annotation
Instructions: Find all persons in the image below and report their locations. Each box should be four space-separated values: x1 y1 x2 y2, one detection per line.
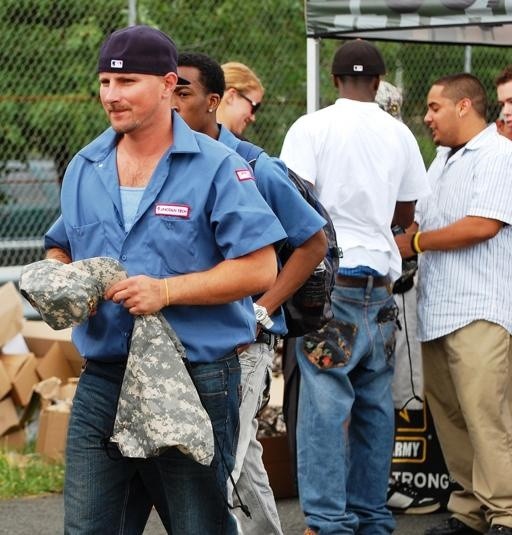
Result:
270 75 417 380
41 25 289 534
280 36 433 534
169 52 329 533
495 65 511 141
215 61 263 136
392 72 511 533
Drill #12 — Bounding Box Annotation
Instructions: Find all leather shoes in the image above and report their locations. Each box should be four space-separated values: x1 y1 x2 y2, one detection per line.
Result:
424 518 480 535
487 524 512 535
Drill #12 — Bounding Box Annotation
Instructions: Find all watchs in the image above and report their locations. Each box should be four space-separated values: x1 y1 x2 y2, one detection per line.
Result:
250 302 275 330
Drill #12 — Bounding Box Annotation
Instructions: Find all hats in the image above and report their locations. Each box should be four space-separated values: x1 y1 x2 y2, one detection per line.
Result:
332 39 385 75
21 258 127 329
375 80 403 123
98 24 191 85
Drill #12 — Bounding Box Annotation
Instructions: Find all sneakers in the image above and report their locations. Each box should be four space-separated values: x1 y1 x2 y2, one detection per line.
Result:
387 478 440 516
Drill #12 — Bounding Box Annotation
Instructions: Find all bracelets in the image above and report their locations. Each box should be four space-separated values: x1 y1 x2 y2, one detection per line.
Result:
410 232 417 254
414 231 423 253
164 278 171 307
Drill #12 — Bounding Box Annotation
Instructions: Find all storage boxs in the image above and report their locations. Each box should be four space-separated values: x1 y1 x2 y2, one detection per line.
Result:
0 282 85 464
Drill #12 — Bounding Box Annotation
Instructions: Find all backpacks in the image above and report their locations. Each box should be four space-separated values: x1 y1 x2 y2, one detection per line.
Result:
236 142 338 339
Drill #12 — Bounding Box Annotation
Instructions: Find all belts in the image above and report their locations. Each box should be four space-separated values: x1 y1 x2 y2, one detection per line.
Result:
335 274 391 288
256 333 277 350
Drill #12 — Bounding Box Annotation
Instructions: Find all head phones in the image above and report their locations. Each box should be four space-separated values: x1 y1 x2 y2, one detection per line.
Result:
391 225 420 293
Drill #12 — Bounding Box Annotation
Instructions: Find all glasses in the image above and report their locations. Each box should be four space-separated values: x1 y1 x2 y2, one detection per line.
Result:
230 87 261 113
